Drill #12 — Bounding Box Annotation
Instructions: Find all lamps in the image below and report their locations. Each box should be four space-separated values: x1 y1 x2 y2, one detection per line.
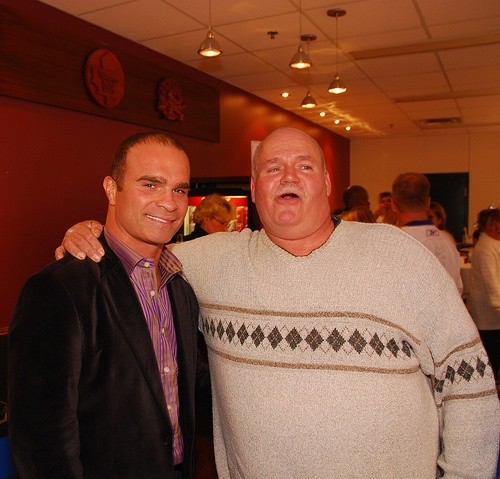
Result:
327 8 347 94
197 0 223 57
300 34 317 108
290 0 313 69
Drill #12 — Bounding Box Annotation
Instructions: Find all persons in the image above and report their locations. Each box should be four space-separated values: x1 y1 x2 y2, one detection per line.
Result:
0 135 220 479
54 127 500 479
184 169 500 397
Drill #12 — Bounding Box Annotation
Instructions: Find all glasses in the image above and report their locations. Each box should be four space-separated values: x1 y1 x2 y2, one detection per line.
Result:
213 218 231 228
